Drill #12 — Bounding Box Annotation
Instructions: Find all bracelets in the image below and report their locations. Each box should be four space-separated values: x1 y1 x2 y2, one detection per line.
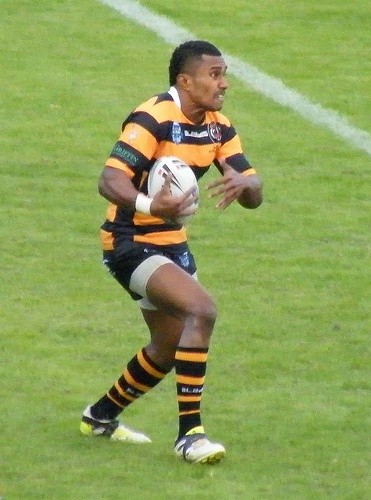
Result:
135 192 151 216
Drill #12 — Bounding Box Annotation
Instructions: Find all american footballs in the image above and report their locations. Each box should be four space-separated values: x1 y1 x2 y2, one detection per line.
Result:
147 155 200 216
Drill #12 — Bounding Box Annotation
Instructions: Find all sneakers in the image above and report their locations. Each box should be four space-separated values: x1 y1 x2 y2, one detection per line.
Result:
173 425 226 465
79 404 152 443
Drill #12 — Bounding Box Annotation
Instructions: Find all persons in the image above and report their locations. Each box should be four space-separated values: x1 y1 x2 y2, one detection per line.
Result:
80 41 264 466
